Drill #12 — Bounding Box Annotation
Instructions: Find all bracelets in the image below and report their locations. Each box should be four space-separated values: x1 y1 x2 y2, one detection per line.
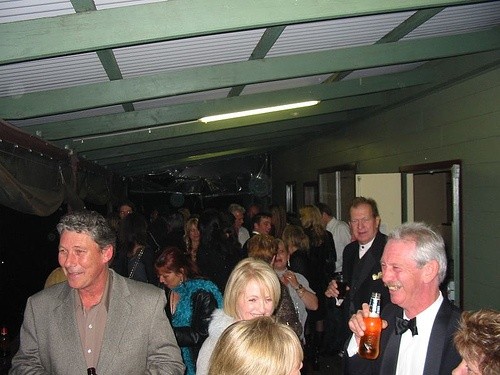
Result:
297 289 307 299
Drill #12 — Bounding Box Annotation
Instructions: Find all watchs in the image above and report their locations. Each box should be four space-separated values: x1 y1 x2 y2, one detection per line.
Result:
294 284 303 291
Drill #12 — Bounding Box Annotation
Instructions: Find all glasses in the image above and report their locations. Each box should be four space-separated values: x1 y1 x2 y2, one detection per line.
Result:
349 217 376 224
120 209 134 216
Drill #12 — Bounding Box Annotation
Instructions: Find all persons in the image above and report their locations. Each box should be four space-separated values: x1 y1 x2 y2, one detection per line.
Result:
44 200 354 321
327 196 391 313
8 210 187 375
343 222 463 375
273 238 318 329
153 246 223 375
299 207 337 370
208 316 303 375
247 234 303 336
452 309 500 375
196 257 281 375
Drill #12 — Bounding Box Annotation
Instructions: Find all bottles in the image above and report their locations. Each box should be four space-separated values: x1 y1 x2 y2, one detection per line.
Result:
358 292 381 359
337 274 347 299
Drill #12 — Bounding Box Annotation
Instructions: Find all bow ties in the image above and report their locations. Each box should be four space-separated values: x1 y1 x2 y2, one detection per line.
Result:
395 316 418 336
